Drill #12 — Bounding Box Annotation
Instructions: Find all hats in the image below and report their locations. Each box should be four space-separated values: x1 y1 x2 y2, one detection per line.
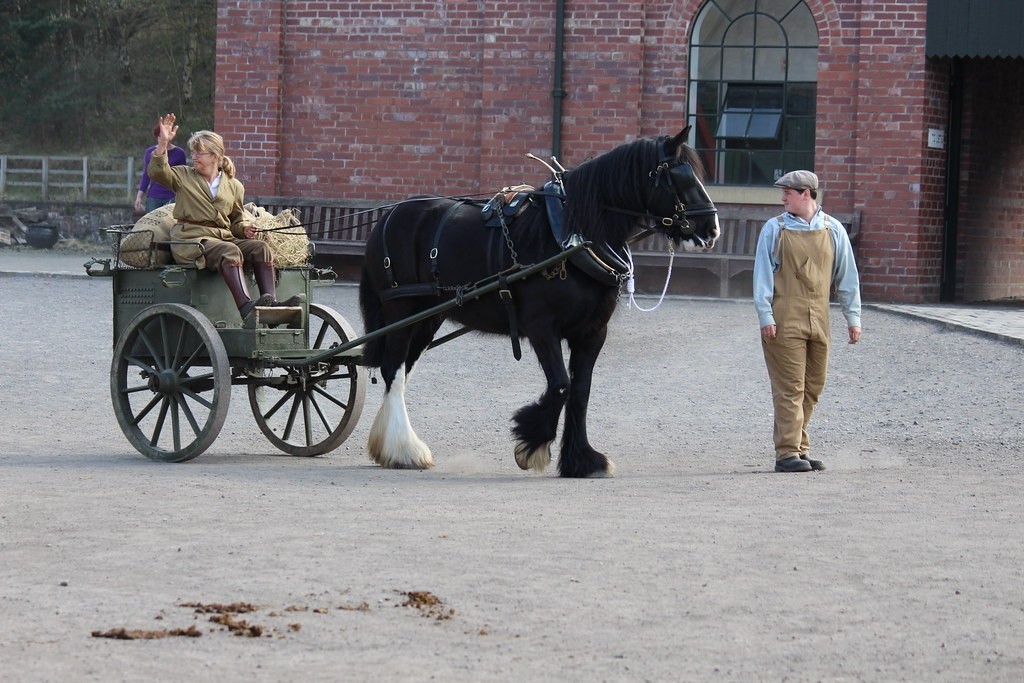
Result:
773 170 819 190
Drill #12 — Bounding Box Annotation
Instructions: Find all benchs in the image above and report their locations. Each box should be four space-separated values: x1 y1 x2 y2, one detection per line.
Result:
246 197 861 300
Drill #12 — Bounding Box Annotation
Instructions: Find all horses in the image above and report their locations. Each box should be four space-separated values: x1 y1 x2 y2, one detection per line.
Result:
359 124 720 479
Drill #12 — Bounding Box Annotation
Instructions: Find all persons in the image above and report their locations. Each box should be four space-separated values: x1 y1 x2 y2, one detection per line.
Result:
147 113 302 330
753 170 862 472
134 125 186 214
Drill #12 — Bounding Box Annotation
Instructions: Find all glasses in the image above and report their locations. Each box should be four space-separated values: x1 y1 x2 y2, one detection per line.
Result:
190 150 214 158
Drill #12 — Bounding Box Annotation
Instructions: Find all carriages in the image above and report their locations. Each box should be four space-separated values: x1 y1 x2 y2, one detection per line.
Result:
82 124 722 479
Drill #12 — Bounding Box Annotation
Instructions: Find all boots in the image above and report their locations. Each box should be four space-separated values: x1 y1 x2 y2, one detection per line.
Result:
221 266 274 323
254 263 302 306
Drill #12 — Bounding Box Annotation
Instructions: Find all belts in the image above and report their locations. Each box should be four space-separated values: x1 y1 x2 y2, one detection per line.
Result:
177 219 219 228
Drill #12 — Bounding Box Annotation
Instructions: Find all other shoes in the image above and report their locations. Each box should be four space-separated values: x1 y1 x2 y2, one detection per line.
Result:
800 454 825 470
775 455 812 472
